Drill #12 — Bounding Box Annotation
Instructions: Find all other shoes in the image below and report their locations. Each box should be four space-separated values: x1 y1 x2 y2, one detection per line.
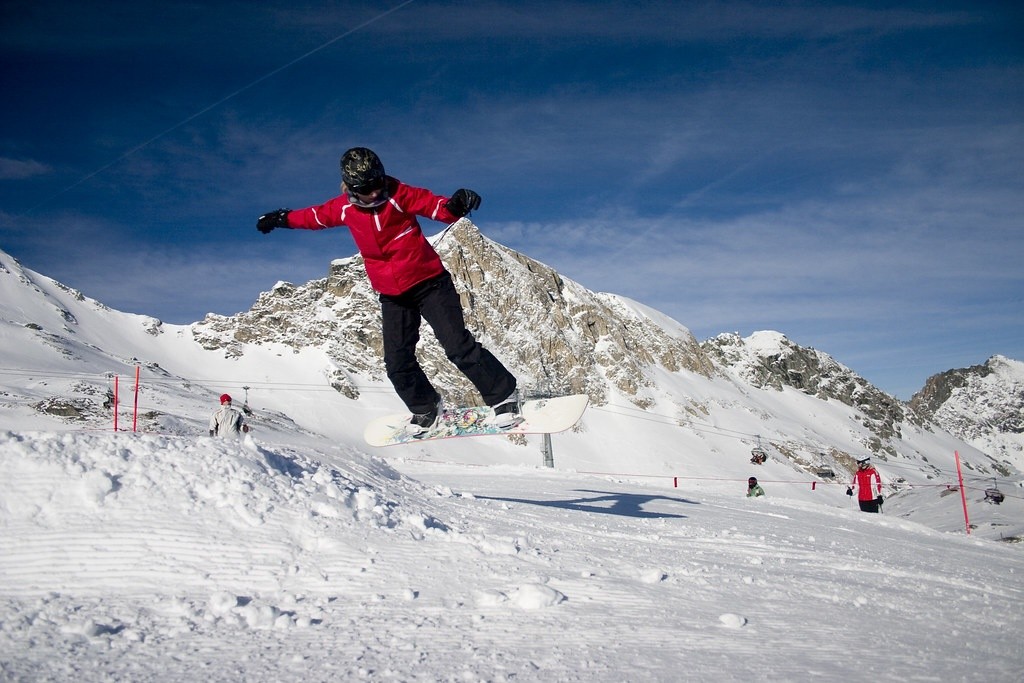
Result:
406 398 441 436
492 386 524 430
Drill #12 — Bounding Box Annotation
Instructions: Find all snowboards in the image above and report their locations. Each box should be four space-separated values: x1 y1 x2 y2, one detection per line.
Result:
362 393 590 448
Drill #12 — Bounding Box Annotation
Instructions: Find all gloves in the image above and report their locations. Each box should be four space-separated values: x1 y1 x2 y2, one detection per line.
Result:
257 208 292 234
846 487 853 496
445 189 481 218
877 496 883 507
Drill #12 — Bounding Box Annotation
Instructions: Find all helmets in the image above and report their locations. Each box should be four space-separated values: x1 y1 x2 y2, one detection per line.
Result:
340 147 385 195
856 454 870 469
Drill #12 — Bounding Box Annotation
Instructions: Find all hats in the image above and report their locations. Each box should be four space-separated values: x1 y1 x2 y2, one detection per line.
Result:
220 394 231 404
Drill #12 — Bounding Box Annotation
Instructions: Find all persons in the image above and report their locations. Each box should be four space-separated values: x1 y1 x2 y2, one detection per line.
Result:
210 394 248 436
846 457 884 513
751 452 766 465
747 477 764 497
255 148 523 439
